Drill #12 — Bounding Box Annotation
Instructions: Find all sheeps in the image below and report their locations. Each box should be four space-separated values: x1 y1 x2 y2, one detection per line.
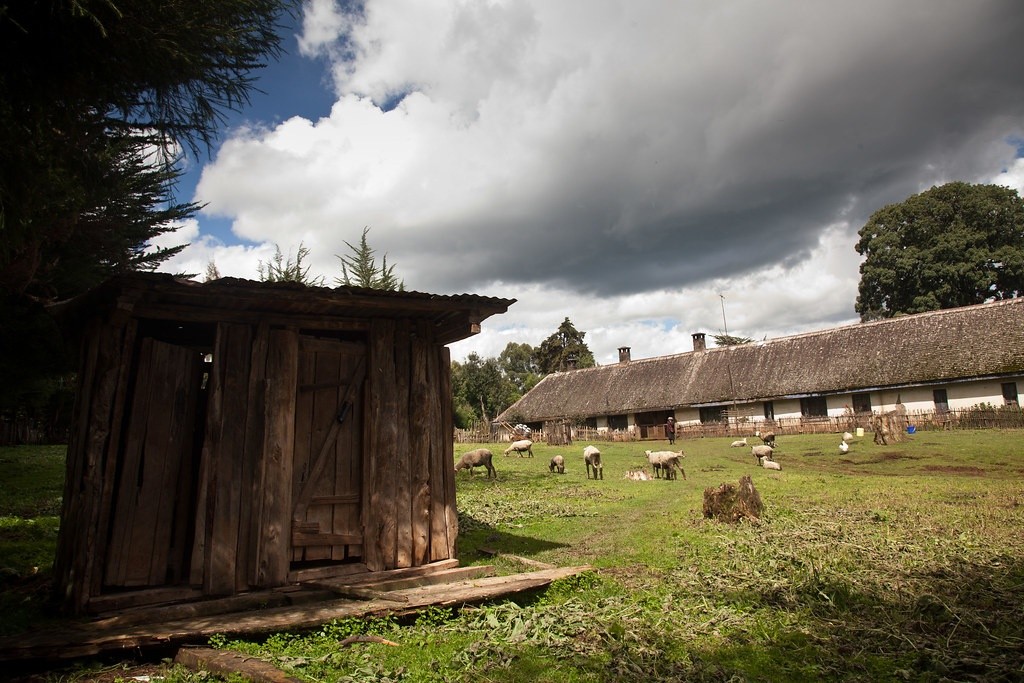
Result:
504 440 534 458
644 449 687 481
548 454 565 474
838 441 849 454
756 430 776 448
751 445 774 466
454 448 497 479
583 444 604 480
730 437 748 448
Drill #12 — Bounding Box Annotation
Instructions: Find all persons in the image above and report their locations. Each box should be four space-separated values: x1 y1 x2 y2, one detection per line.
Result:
666 417 675 445
